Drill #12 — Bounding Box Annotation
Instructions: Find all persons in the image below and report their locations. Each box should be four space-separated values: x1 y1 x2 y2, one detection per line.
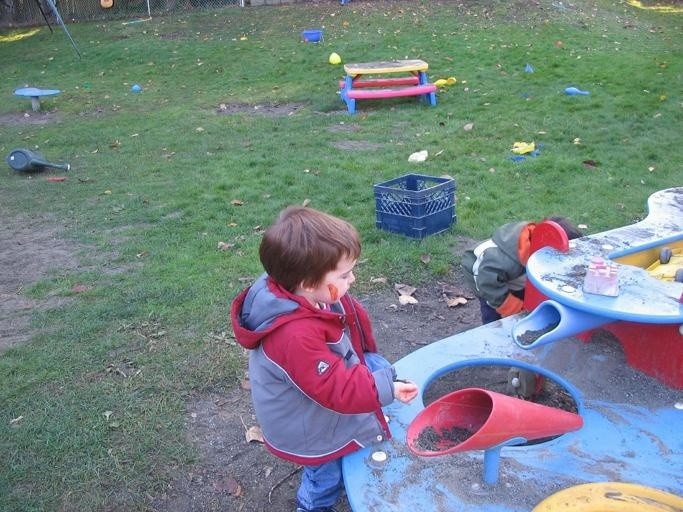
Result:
229 205 418 511
459 216 584 325
